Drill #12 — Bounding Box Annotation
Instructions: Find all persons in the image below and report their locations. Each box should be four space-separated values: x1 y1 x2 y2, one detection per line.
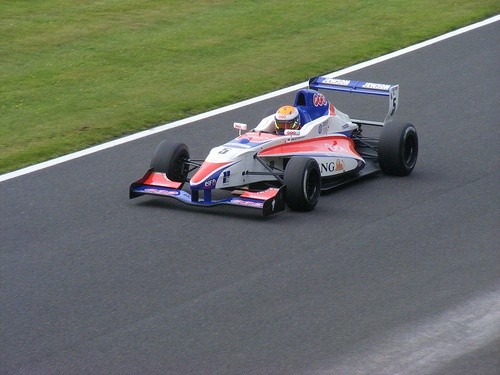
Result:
273 106 301 135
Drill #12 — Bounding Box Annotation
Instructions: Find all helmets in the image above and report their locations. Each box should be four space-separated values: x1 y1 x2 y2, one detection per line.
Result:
274 106 300 135
